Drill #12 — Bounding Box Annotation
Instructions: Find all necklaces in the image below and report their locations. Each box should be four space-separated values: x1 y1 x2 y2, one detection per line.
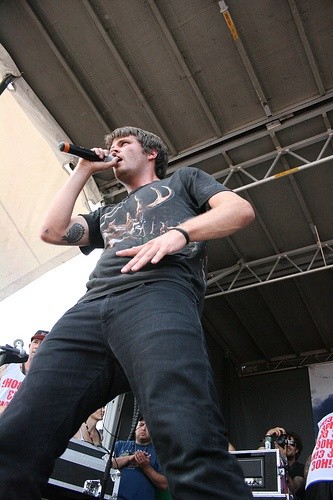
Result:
85 421 101 446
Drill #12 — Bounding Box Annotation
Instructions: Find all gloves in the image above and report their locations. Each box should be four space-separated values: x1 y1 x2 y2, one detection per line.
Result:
0 344 29 366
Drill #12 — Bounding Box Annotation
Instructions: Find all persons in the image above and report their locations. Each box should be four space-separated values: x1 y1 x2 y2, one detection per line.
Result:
257 427 306 498
74 406 105 448
111 414 168 500
0 330 51 416
0 126 256 500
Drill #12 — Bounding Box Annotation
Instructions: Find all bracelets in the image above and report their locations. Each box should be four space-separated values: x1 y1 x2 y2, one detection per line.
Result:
170 228 189 245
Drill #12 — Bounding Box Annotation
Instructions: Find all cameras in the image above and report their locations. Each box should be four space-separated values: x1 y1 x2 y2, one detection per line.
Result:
275 430 287 448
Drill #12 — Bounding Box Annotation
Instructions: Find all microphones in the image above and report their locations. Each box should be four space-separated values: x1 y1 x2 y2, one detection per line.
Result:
0 346 26 358
58 142 114 162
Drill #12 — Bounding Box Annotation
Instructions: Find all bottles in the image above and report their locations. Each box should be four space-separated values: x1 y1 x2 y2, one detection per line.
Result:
264 434 272 450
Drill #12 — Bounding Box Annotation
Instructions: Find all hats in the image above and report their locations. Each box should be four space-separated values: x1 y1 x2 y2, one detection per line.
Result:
31 330 49 342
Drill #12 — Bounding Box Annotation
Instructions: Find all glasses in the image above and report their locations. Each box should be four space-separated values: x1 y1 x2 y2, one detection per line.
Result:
285 441 296 447
